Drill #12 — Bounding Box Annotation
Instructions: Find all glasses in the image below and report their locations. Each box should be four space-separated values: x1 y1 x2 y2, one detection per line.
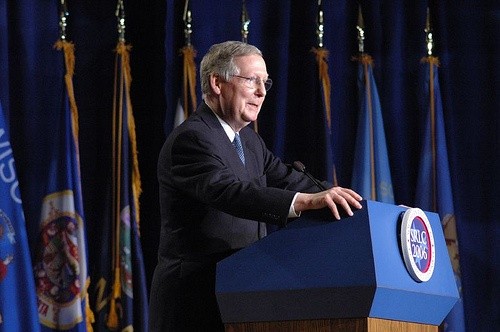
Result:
229 74 273 92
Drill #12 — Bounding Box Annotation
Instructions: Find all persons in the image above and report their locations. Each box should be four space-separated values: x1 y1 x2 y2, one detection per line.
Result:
148 40 363 332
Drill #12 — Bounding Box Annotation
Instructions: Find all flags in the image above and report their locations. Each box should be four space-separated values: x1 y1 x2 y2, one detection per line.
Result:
414 57 465 332
173 44 197 128
349 53 394 204
95 43 148 332
33 40 95 332
0 99 40 332
309 47 337 189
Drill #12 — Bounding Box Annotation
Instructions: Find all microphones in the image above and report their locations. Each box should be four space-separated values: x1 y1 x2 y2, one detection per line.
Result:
292 161 327 191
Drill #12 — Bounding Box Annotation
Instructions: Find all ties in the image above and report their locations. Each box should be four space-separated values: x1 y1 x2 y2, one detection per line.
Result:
234 133 246 167
